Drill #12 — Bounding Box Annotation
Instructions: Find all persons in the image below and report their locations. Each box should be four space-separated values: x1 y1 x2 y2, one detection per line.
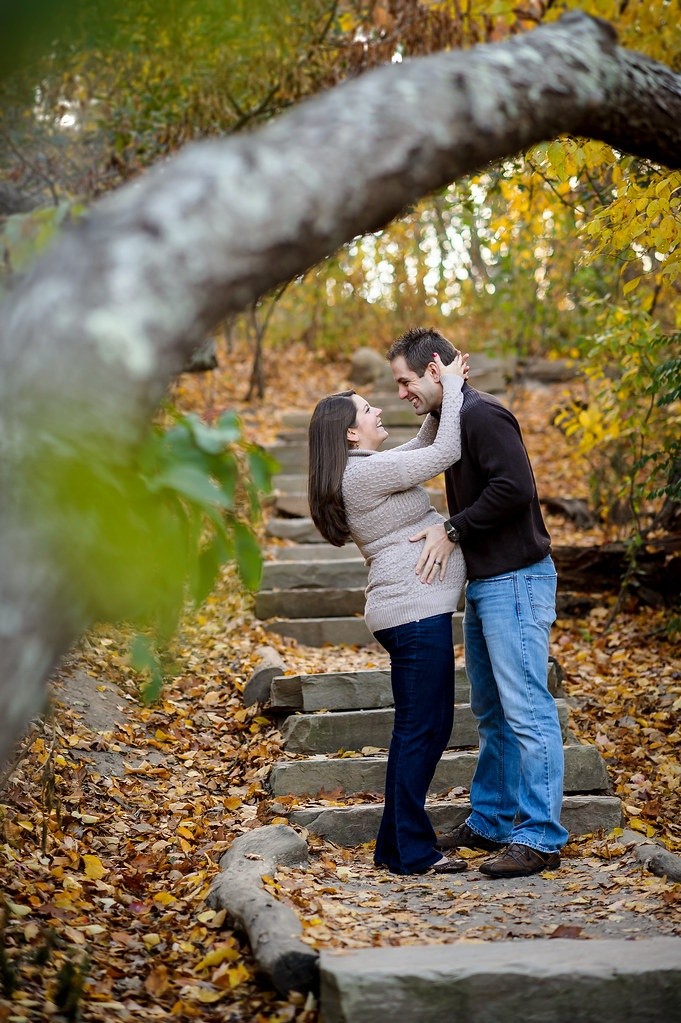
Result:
308 352 469 874
386 328 569 878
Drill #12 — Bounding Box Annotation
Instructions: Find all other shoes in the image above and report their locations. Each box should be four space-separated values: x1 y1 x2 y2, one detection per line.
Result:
427 856 469 873
373 846 397 866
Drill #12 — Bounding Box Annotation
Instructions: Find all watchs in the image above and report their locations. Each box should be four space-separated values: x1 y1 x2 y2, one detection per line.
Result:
444 521 459 543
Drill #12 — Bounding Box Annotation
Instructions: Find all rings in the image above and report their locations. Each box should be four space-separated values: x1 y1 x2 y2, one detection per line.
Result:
434 561 441 565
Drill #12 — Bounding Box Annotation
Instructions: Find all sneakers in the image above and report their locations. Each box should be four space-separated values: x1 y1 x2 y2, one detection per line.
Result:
480 844 562 878
435 821 504 851
